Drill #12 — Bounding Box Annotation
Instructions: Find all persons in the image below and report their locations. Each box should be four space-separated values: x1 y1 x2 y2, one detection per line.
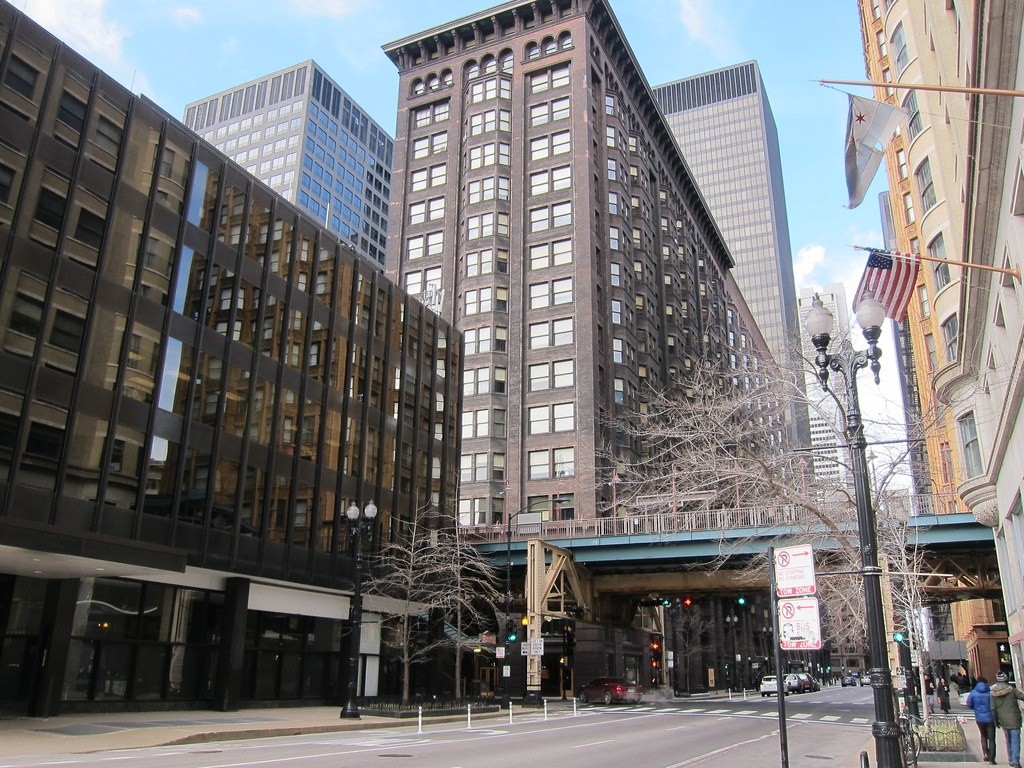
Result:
950 673 987 691
917 674 951 714
990 672 1024 768
966 678 997 764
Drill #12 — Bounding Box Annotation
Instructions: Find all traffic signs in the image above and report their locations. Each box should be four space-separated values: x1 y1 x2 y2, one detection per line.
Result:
521 642 529 655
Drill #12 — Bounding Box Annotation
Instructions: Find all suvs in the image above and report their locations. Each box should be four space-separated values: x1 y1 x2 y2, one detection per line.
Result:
782 674 804 694
760 676 789 697
860 676 871 686
798 673 819 692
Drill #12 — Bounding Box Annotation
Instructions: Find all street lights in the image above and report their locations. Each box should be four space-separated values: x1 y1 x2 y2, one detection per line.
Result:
339 497 377 719
803 286 901 768
502 498 571 709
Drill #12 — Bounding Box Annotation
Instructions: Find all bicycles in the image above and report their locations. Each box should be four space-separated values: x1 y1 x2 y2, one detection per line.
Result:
898 712 926 768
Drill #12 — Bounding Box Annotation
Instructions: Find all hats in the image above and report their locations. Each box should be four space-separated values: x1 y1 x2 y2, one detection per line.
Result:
976 676 987 684
995 670 1008 682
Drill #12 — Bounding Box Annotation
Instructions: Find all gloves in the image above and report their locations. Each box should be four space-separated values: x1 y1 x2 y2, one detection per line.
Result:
994 721 1001 729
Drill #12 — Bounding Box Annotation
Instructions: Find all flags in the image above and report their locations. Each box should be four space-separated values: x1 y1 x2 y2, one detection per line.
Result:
852 247 921 323
843 94 909 209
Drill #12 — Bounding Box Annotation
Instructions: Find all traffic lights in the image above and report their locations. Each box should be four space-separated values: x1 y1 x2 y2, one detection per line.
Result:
684 600 705 607
893 615 902 642
738 598 751 605
652 635 661 662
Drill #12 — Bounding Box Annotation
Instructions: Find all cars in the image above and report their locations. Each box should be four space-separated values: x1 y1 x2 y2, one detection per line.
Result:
958 682 1016 706
842 677 856 687
577 677 643 705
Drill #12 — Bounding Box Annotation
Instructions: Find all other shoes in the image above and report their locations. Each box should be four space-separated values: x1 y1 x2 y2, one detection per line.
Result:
944 709 949 713
985 756 996 765
1010 762 1021 768
929 712 931 714
931 709 934 713
